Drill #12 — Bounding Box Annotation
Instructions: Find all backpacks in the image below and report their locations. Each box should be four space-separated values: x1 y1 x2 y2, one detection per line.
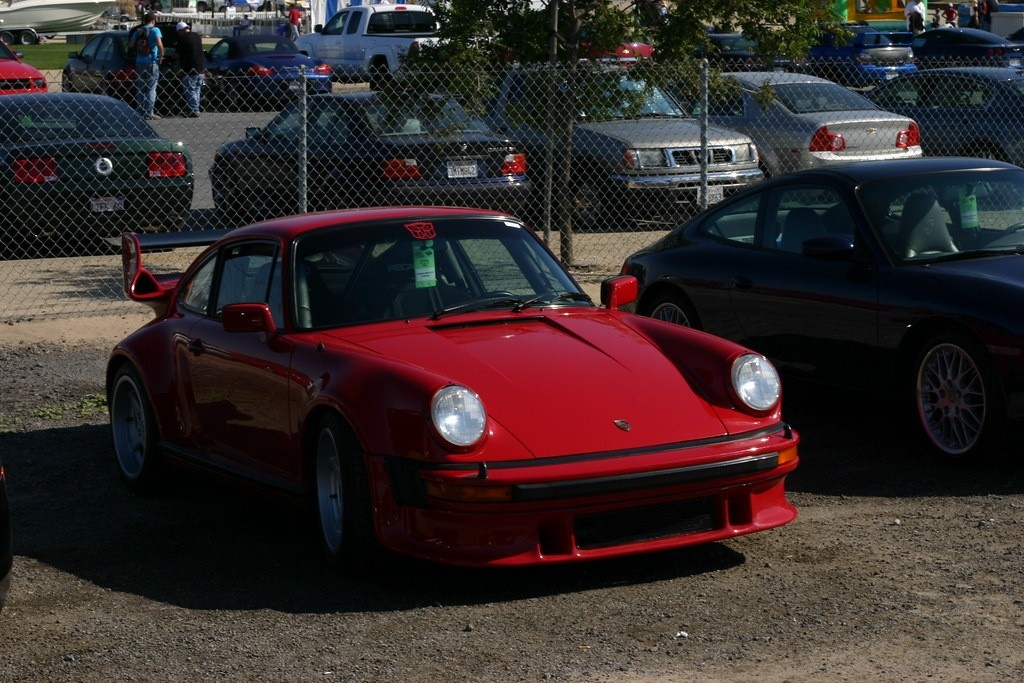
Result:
135 26 156 56
987 0 999 13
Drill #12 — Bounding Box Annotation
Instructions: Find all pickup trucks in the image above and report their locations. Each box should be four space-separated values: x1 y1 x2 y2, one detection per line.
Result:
293 4 519 81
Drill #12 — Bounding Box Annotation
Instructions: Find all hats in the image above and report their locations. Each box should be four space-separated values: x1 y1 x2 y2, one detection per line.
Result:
177 21 188 31
948 2 953 7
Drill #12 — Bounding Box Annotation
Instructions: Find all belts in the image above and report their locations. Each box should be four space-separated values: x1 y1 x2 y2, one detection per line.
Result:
945 21 955 22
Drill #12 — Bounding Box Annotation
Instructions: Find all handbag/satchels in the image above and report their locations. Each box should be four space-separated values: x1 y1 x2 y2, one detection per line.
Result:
913 26 925 36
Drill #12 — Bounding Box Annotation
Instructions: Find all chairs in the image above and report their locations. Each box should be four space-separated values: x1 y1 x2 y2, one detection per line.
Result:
338 15 348 34
782 208 825 256
390 277 476 316
257 260 369 326
896 192 960 259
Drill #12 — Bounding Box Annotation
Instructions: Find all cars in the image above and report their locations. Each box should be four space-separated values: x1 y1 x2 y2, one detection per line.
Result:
202 35 333 112
493 15 1024 100
104 206 803 606
464 63 766 232
0 37 47 95
62 22 235 118
862 68 1023 207
209 93 536 229
619 156 1023 490
632 71 921 182
0 94 194 247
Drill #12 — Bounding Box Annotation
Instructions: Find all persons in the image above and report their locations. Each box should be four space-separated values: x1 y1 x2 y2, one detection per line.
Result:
233 15 250 37
175 22 205 118
130 13 164 120
289 4 302 42
904 0 999 36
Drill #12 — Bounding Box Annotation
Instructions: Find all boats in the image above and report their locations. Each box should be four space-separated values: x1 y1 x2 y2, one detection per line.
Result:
0 1 117 30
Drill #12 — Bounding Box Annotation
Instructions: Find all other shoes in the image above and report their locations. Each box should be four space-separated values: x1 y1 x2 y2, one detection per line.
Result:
184 110 200 118
144 112 161 120
